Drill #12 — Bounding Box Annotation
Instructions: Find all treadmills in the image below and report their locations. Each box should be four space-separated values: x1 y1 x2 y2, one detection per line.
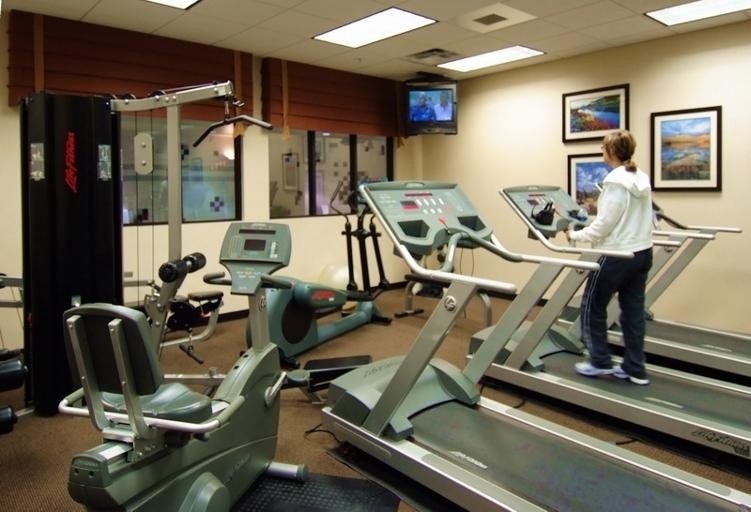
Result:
466 185 750 459
320 180 751 512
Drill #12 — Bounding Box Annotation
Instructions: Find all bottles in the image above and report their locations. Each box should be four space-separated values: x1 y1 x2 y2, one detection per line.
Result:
577 204 587 232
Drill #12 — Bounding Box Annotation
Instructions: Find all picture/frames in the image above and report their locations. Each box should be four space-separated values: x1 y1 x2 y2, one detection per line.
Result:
567 152 614 221
560 83 630 143
650 105 722 192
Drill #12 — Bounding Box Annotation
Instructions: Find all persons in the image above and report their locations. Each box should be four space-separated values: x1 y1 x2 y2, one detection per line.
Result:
566 131 653 385
410 90 452 121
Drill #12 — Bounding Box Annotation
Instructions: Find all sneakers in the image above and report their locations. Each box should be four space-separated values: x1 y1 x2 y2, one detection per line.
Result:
612 364 650 386
577 363 616 376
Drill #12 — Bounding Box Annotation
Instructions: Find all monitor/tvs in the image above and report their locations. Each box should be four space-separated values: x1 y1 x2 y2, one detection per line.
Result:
403 81 457 135
